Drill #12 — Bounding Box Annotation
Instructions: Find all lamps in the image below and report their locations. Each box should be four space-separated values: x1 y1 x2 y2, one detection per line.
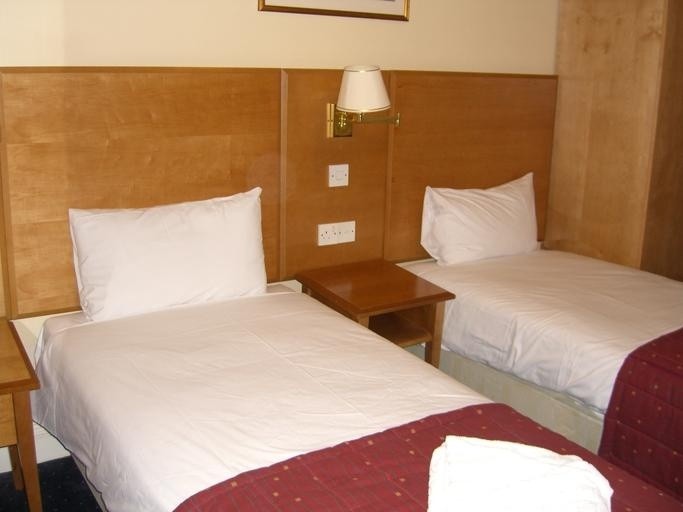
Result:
325 65 401 138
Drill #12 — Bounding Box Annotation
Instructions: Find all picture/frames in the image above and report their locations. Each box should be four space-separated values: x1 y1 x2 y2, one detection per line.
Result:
258 0 412 21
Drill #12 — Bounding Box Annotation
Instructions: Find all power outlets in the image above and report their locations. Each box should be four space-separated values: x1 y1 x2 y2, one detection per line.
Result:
317 164 358 248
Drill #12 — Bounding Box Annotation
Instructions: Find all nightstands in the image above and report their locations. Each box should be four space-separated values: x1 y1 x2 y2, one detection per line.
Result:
295 258 456 373
1 316 40 512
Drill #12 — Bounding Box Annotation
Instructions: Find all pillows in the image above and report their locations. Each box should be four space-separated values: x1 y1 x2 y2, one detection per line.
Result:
68 186 269 324
414 172 548 265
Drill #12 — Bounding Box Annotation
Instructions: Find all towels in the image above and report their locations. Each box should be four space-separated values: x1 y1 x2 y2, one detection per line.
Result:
423 432 613 511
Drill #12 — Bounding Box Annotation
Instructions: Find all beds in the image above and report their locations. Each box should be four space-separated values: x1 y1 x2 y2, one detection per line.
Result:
13 182 683 511
391 170 683 492
